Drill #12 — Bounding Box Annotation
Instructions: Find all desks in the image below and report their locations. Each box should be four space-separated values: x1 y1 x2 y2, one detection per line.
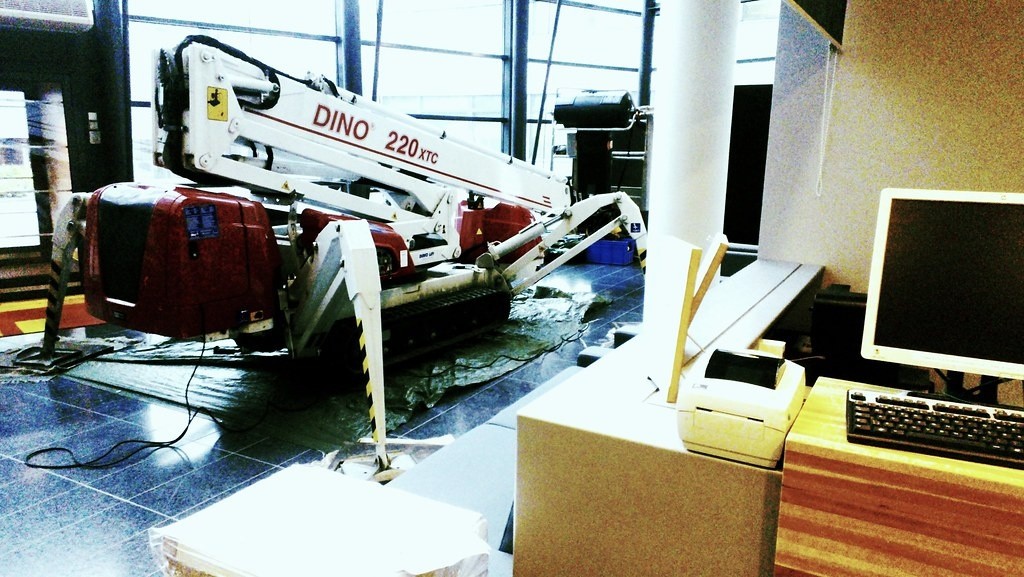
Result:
513 260 825 577
775 376 1023 576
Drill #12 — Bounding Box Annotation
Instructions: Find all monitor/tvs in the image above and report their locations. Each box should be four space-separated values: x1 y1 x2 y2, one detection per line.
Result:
860 187 1024 380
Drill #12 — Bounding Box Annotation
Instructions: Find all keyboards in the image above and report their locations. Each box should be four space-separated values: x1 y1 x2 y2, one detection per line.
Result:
846 388 1024 470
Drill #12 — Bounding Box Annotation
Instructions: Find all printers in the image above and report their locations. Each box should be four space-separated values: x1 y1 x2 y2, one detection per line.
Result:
678 339 805 467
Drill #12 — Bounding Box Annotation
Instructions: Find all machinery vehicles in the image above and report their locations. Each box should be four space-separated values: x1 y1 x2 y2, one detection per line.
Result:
76 35 655 489
